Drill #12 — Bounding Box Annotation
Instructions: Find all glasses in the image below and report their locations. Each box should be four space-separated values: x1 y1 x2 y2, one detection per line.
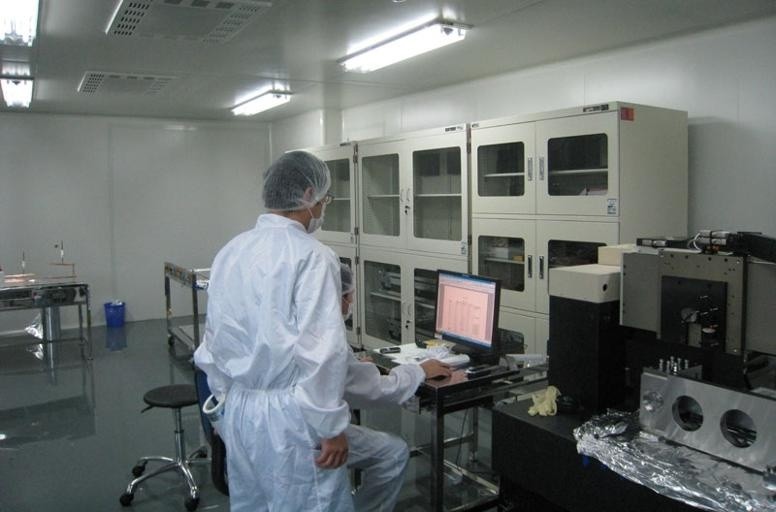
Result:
324 194 335 206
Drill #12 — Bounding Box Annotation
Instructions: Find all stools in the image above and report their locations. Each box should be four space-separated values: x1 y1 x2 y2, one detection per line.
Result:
119 384 213 511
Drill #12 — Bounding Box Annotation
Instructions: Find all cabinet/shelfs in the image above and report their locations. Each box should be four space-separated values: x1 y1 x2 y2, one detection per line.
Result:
357 250 469 348
287 141 355 348
472 103 619 354
0 280 91 372
359 120 471 247
164 261 212 371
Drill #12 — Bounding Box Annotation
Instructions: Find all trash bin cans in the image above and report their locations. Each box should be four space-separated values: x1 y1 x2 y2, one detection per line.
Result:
105 301 125 327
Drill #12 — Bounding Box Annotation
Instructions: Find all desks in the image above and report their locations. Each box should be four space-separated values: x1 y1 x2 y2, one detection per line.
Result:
350 337 551 512
492 378 776 511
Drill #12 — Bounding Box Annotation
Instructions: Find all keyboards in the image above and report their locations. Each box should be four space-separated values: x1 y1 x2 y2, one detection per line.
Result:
370 355 401 371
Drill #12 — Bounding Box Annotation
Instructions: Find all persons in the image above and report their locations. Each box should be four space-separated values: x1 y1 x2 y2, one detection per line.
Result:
194 151 355 512
340 263 451 512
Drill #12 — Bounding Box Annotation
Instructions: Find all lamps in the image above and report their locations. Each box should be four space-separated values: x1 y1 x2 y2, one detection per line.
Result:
229 90 291 116
0 0 40 49
0 76 34 109
336 18 474 74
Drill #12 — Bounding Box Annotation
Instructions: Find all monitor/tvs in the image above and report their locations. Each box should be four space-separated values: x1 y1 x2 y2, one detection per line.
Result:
435 269 501 358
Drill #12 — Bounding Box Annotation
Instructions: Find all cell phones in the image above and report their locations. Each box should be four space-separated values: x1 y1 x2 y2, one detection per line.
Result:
381 347 402 353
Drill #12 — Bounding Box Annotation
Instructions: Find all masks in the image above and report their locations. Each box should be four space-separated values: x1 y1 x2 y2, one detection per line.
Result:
307 202 327 234
342 296 354 322
